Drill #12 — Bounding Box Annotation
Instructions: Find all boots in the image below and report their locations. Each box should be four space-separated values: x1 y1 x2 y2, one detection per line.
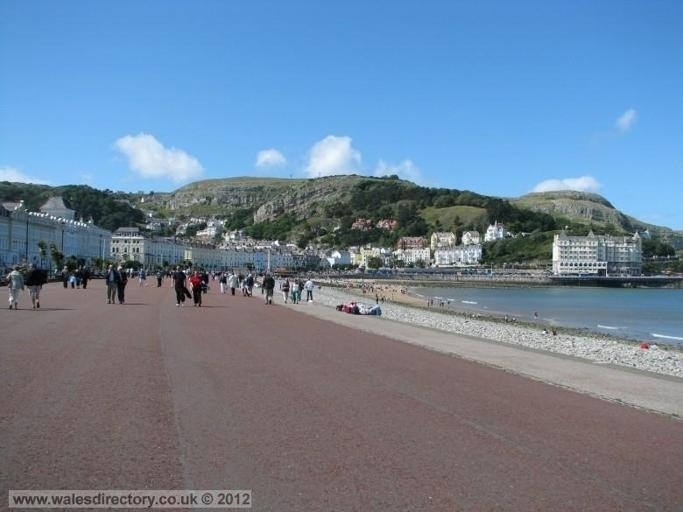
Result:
32 300 40 308
106 301 125 304
264 299 272 305
9 303 18 310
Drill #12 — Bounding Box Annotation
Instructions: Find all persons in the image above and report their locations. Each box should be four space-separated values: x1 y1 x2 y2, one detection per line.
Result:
127 263 314 308
68 274 76 288
6 265 25 309
81 265 91 289
61 265 70 288
117 266 128 304
105 264 122 305
25 262 43 310
362 283 452 307
75 269 81 289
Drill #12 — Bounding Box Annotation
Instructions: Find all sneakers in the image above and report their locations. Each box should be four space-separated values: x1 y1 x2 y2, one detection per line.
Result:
176 302 201 307
